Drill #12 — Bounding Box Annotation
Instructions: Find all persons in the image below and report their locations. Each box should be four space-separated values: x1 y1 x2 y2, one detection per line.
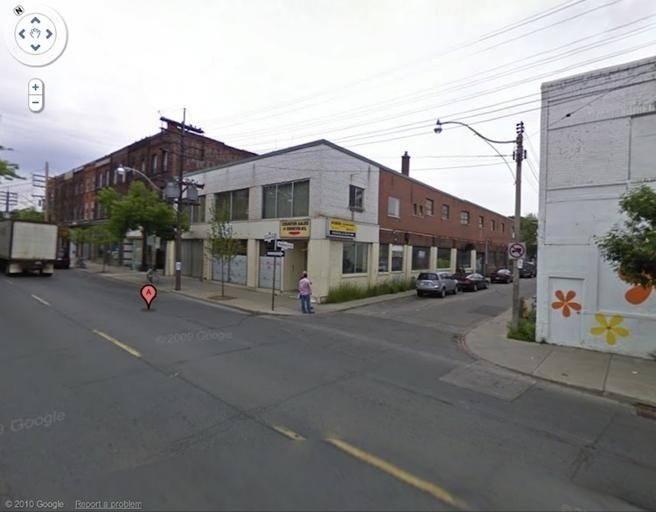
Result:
298 271 313 309
299 274 314 313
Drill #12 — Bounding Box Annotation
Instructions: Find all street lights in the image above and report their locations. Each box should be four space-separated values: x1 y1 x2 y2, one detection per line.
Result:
433 116 522 335
115 162 181 291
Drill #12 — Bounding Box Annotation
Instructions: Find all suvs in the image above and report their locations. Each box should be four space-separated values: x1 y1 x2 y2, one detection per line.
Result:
414 271 458 298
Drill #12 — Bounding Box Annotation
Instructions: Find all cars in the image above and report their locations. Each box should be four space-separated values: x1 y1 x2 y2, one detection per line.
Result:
452 271 490 292
489 267 512 284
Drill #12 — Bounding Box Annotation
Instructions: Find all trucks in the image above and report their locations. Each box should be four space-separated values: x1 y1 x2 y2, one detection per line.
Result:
0 217 61 280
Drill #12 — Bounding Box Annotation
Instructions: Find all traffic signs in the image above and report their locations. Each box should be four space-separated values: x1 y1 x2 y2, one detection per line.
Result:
264 250 285 257
276 240 295 251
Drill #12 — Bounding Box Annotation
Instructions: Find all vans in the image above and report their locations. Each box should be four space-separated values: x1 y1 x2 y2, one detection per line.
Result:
519 261 536 279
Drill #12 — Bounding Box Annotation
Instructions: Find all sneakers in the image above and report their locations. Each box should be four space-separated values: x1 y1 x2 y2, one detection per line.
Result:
301 306 315 314
